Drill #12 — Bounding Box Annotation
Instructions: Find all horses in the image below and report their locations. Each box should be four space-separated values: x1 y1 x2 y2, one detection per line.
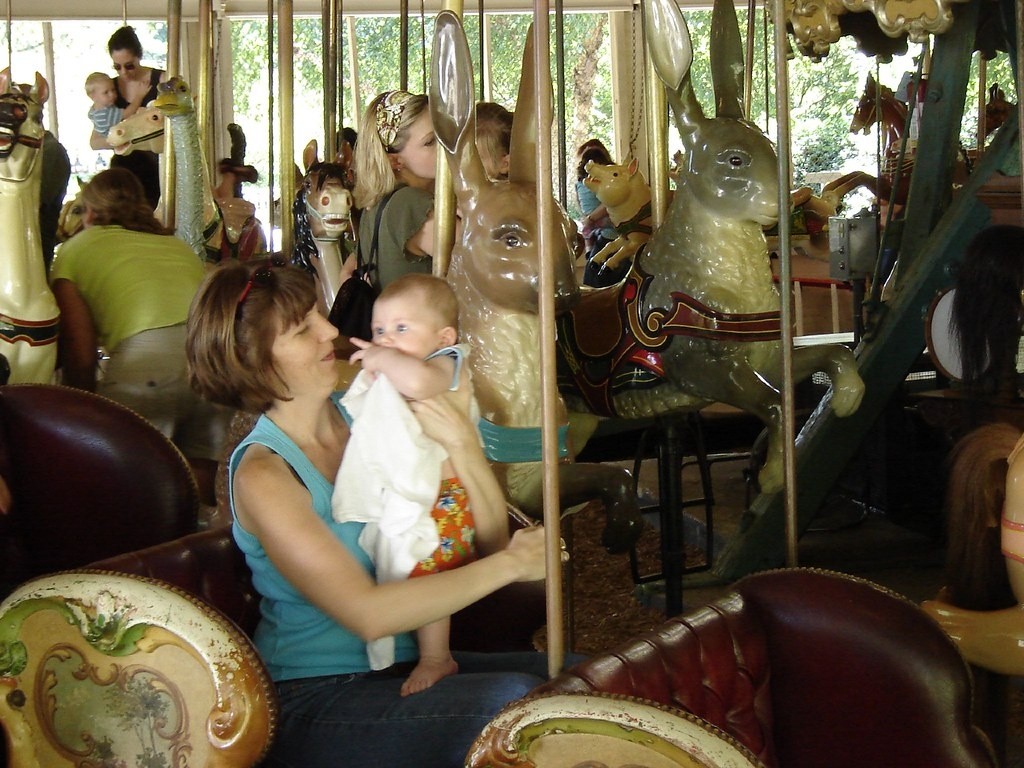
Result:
820 71 1020 206
288 140 355 319
920 222 1023 677
107 99 167 226
0 66 68 388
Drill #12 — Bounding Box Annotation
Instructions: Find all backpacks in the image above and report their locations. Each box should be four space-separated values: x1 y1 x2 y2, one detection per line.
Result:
326 184 407 340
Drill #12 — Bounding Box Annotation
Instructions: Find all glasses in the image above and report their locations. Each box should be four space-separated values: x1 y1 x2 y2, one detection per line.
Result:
113 57 138 70
238 253 287 305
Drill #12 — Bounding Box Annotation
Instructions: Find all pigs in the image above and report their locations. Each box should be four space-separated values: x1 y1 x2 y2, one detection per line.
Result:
583 151 686 277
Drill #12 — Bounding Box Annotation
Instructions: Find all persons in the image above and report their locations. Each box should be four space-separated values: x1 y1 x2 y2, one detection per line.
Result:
37 130 72 287
326 89 462 341
475 101 514 182
336 127 365 242
332 274 487 697
84 73 152 151
89 28 166 214
50 168 209 440
575 139 633 289
184 261 597 768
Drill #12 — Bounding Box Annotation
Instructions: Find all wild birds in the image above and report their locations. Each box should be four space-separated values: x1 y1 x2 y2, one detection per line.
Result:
155 75 267 268
